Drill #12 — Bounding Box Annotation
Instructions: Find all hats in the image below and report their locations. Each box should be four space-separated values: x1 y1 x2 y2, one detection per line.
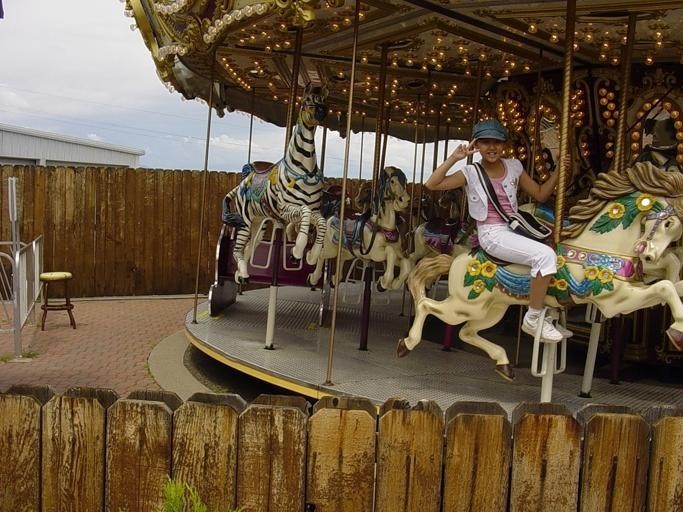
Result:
471 118 510 142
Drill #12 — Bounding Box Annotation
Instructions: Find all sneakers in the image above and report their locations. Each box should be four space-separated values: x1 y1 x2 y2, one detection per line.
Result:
521 312 563 344
545 315 573 339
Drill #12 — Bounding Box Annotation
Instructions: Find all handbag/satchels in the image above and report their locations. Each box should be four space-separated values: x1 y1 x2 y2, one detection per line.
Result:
508 210 552 241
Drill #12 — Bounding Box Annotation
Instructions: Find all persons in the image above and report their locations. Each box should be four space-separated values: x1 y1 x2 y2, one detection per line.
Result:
423 116 574 345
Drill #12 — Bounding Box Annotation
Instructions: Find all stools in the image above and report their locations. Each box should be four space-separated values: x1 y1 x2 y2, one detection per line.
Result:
38 270 79 330
